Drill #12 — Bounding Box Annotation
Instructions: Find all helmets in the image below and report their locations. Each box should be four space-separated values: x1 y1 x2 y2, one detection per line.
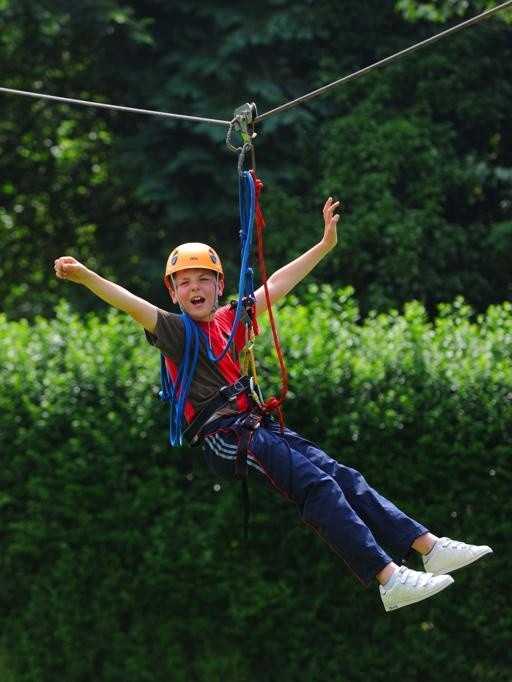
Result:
163 240 225 289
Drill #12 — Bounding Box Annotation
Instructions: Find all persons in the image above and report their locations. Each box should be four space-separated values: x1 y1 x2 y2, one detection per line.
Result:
53 197 493 613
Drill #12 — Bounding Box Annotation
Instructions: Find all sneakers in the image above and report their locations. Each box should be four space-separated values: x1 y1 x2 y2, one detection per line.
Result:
378 565 455 613
421 536 494 575
